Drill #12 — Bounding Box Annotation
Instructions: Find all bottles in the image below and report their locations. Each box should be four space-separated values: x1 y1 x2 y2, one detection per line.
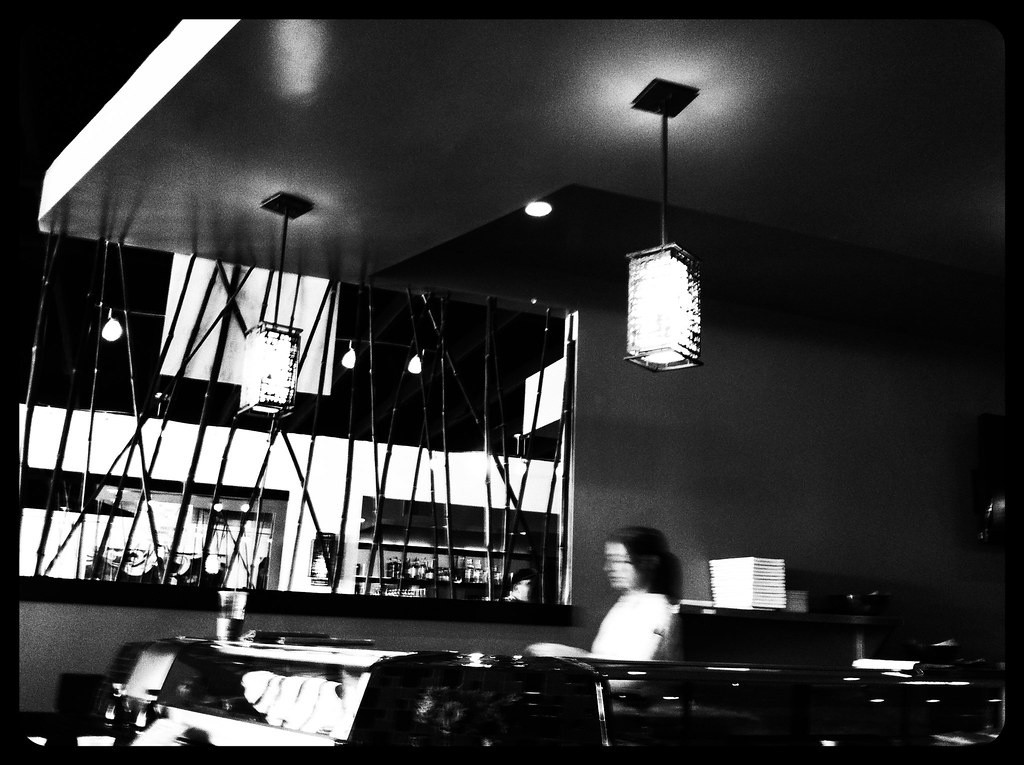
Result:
385 588 426 598
386 557 501 584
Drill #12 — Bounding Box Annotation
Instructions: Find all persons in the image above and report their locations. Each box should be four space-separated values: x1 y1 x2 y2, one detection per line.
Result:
525 524 685 692
503 532 556 605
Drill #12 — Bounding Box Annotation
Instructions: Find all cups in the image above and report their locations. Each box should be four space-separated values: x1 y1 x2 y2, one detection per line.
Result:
217 590 249 641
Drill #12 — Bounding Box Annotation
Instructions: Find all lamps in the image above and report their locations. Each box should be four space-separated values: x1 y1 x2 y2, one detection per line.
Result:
232 191 319 418
616 76 706 372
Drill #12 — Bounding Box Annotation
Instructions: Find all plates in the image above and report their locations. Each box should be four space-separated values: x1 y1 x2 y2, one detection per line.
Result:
709 556 808 612
